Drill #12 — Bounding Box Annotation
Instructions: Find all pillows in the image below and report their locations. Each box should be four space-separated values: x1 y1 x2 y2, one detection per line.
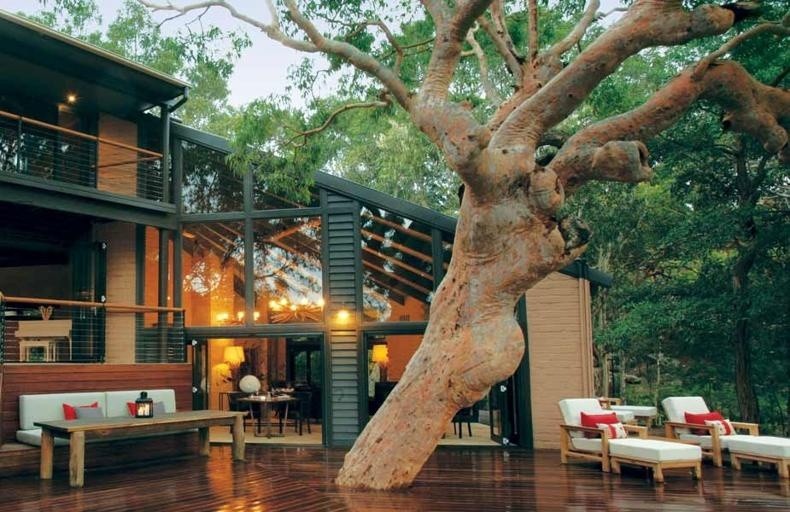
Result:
63 401 98 420
127 402 135 416
153 401 165 415
579 411 628 439
73 407 105 419
685 410 738 436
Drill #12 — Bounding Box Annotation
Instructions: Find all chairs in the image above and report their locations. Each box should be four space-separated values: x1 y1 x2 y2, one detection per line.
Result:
607 398 658 429
557 398 703 484
661 396 790 479
598 399 638 425
441 402 476 439
278 391 313 436
368 381 398 416
227 391 262 434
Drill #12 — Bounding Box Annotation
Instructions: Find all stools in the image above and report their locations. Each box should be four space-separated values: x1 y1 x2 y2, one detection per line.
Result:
19 341 55 363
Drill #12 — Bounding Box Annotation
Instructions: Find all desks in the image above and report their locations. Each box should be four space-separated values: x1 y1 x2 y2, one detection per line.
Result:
32 410 249 487
15 319 73 362
237 397 301 437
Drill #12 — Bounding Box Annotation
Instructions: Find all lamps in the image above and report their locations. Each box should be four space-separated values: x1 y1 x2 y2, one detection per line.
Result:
224 346 246 383
372 345 389 362
94 239 109 249
135 392 153 418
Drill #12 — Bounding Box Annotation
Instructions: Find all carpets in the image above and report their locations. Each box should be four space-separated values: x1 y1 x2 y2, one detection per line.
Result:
209 418 518 447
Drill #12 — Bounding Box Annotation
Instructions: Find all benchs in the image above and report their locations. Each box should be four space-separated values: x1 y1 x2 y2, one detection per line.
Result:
1 361 201 476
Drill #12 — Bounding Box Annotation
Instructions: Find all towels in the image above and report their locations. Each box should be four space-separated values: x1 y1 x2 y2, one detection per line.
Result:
40 305 52 320
